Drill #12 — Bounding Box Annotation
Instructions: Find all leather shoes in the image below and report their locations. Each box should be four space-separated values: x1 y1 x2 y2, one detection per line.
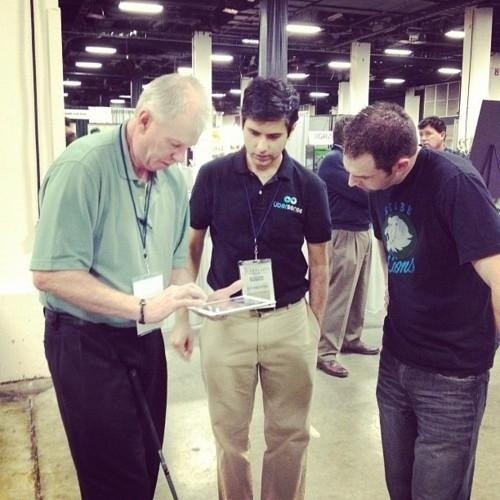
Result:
317 358 348 377
341 339 380 355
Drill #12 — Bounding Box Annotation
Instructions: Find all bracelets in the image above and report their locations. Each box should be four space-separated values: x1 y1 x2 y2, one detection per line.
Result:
139 297 147 325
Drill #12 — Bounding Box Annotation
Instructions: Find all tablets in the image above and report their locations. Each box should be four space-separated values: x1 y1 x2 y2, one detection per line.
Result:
186 294 277 318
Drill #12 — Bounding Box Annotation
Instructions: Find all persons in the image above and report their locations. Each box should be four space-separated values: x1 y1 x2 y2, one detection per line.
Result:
29 72 244 500
317 114 381 379
342 100 500 499
419 114 467 161
171 79 334 500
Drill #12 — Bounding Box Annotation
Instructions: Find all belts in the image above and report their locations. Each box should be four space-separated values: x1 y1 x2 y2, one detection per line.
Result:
252 300 302 313
